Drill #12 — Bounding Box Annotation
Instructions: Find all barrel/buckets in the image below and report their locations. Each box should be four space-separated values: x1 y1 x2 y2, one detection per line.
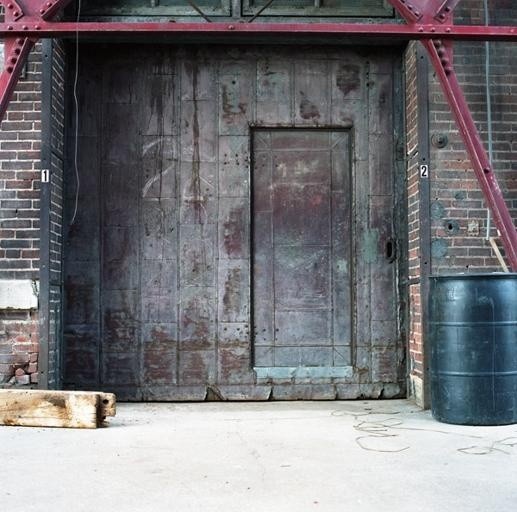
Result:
427 270 516 426
